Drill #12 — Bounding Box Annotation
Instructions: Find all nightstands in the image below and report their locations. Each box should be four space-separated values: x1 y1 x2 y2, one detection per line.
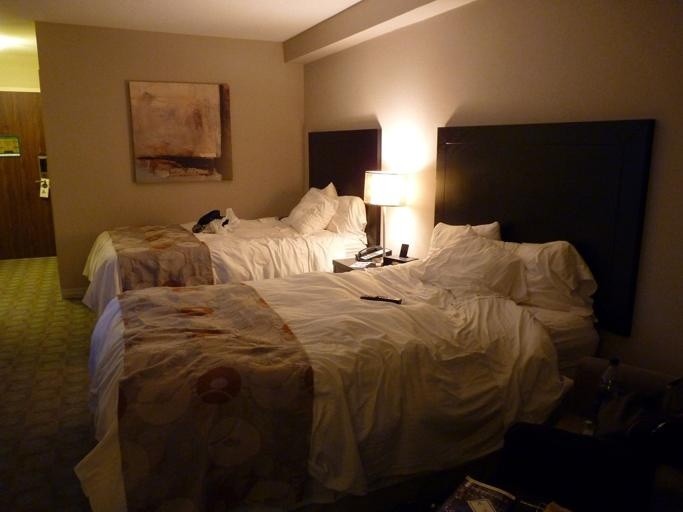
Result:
333 258 382 273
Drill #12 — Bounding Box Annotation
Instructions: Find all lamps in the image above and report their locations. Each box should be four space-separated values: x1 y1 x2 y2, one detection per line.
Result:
364 170 415 266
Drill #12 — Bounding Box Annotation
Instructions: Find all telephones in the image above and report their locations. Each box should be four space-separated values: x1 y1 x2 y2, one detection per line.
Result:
355 246 392 262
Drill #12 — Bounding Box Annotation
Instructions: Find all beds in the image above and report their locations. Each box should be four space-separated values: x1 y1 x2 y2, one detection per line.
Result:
82 182 368 316
74 221 596 512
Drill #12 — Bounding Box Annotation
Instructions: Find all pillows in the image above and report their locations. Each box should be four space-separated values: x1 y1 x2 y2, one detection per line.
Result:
422 221 598 317
281 182 368 236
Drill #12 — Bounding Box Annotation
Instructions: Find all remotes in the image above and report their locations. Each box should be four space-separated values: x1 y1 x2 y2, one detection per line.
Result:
360 294 404 304
